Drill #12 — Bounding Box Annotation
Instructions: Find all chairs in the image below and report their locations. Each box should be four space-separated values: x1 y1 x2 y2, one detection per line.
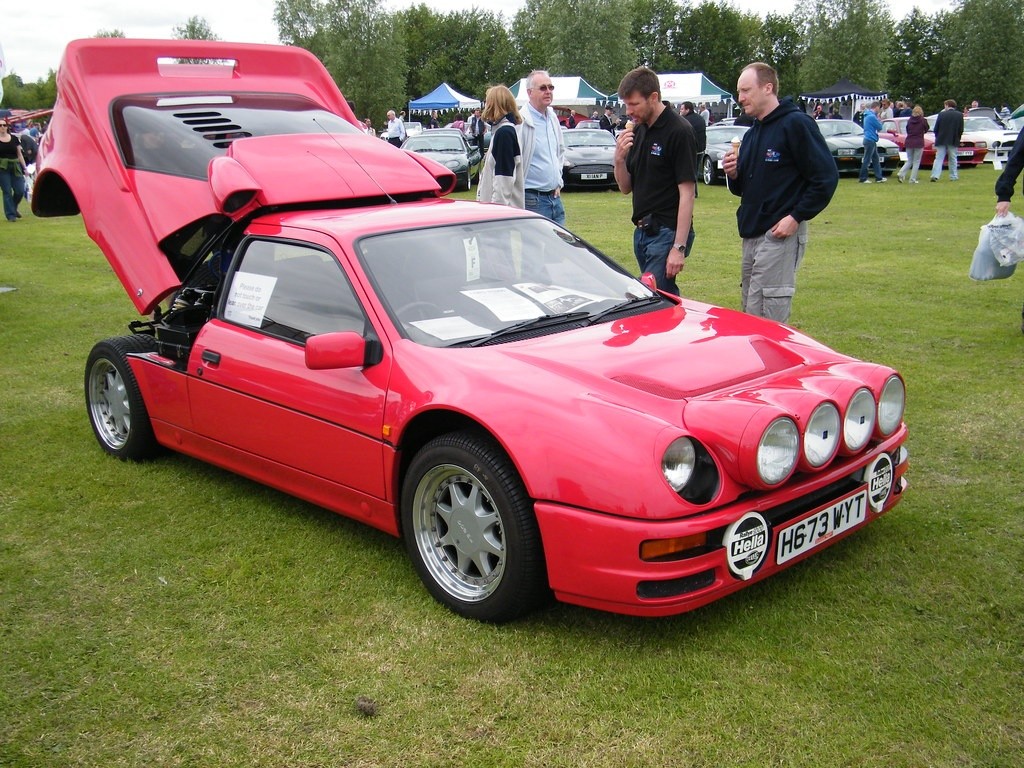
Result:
374 240 468 302
589 136 604 144
412 141 429 149
265 256 345 333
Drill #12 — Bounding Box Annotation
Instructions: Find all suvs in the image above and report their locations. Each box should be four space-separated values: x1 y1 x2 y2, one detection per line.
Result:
964 106 1006 130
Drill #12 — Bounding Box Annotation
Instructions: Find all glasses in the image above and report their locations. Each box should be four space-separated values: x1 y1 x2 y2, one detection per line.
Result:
531 86 555 92
0 124 7 127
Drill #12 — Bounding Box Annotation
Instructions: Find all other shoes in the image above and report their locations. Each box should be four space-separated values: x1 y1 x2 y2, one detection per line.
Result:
897 175 903 183
910 180 919 183
16 211 22 218
876 177 887 183
931 178 936 181
860 180 872 185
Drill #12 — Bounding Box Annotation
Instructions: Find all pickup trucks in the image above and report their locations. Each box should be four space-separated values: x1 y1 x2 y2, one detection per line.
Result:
400 128 481 191
381 122 426 141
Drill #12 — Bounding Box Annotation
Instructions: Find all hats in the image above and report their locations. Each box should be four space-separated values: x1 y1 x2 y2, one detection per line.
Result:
30 128 38 136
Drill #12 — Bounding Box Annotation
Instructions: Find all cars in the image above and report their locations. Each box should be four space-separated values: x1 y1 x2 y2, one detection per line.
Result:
561 129 619 191
815 119 901 179
442 122 492 150
927 117 1021 163
614 122 627 139
877 119 988 168
31 36 909 623
704 117 751 185
574 120 614 137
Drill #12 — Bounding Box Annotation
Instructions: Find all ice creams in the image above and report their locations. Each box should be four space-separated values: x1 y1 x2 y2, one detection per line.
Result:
625 119 633 130
731 136 740 157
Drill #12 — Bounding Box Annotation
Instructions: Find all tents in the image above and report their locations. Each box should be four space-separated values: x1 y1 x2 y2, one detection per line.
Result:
508 77 610 108
409 82 481 128
611 73 732 119
799 79 888 122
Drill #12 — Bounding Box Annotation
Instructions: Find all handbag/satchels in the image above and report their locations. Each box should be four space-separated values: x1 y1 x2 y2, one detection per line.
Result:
23 174 33 202
969 210 1024 281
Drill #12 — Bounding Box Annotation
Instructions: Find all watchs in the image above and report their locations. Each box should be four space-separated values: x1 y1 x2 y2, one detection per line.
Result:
674 244 686 252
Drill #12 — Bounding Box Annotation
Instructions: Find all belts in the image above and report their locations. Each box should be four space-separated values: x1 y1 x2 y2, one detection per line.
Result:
526 188 553 195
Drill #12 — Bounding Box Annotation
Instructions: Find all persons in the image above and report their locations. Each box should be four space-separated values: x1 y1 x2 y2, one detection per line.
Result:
515 71 566 280
348 101 376 136
722 62 839 323
590 106 620 136
812 99 1010 184
680 102 755 198
451 109 487 158
995 126 1024 333
387 110 406 148
569 110 576 128
430 112 439 128
602 303 793 347
477 87 525 279
0 120 51 222
614 68 697 297
